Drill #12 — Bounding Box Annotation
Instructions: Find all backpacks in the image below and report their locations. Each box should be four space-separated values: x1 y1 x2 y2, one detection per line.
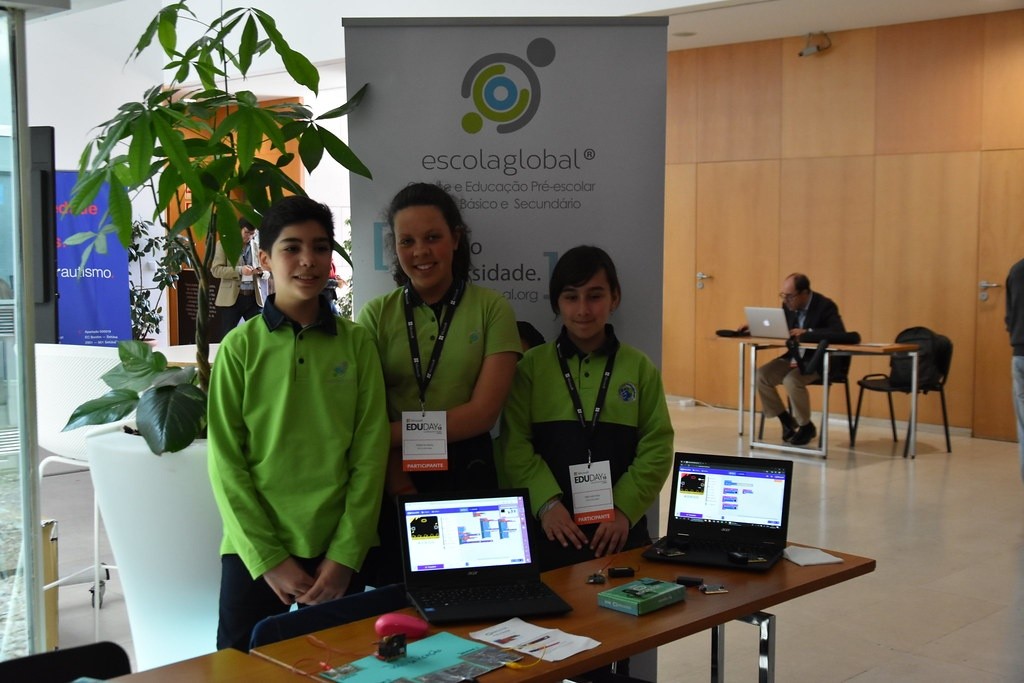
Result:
890 327 940 390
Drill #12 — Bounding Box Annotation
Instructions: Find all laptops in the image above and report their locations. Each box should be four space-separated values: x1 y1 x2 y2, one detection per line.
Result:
743 306 791 339
641 452 794 574
395 487 574 627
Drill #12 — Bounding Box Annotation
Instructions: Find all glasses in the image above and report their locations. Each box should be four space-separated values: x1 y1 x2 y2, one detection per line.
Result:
241 225 257 236
779 291 805 301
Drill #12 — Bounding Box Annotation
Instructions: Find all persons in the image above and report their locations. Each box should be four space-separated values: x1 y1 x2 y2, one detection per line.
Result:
356 182 523 585
500 246 674 572
324 258 348 314
738 273 847 445
206 197 388 653
1005 259 1024 480
211 217 271 341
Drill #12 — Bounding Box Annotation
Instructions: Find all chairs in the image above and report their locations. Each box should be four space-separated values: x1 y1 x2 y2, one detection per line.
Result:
758 329 860 447
0 639 132 683
246 582 409 649
850 326 955 457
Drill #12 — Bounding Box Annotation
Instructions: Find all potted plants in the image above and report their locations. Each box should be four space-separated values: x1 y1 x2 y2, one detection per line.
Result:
58 1 374 677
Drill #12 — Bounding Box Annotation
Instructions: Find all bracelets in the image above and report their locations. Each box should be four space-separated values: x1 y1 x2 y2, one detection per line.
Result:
539 498 557 517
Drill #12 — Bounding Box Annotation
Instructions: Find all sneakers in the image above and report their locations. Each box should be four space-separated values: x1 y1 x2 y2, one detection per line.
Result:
790 422 817 446
776 411 798 442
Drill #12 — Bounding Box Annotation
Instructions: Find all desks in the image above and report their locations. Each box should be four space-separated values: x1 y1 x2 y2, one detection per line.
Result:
102 647 325 683
716 335 923 460
244 538 877 683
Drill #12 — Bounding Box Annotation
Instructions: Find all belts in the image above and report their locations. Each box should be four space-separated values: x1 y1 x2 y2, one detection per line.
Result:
239 289 255 296
